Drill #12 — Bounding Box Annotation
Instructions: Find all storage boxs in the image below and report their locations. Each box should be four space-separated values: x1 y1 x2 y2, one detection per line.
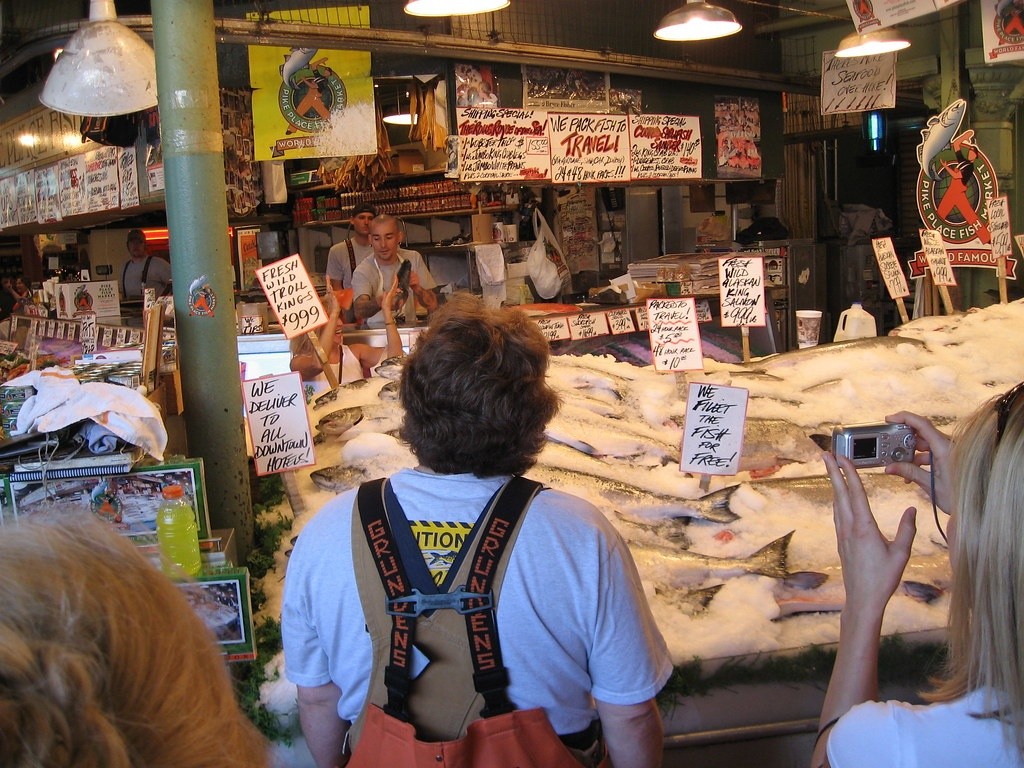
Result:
290 170 321 186
389 149 424 174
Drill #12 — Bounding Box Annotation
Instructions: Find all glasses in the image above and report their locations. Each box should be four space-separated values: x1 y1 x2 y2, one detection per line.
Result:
994 382 1024 446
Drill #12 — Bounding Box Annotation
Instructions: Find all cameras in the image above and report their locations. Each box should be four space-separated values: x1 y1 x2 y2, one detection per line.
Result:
831 421 915 469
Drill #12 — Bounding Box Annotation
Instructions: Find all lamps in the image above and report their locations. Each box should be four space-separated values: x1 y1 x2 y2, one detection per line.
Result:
834 27 911 58
382 104 418 125
654 0 742 41
37 0 160 117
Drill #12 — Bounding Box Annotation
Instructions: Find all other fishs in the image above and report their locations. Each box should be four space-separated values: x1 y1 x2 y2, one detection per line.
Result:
307 312 1006 624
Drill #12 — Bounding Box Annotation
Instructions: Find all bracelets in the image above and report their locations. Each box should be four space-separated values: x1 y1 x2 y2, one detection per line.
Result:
385 321 395 325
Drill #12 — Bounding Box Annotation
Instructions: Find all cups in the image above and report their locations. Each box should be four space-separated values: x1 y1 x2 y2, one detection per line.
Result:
38 277 59 303
235 302 269 334
795 309 822 349
470 214 518 243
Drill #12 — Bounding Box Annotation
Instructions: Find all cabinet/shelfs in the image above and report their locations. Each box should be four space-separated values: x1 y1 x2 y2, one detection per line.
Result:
295 165 517 228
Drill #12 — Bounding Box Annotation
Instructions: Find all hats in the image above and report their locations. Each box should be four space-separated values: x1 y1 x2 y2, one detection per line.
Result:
352 202 376 218
127 230 146 243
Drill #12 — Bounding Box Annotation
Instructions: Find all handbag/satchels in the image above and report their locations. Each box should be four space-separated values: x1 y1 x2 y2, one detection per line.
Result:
526 207 570 299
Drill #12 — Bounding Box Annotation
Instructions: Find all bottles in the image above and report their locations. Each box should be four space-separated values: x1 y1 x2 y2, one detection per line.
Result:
404 284 417 324
156 485 202 579
22 291 56 319
292 180 531 227
833 302 877 343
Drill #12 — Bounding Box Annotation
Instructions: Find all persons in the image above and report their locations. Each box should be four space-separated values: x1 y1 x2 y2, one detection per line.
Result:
810 384 1024 768
325 203 376 324
0 506 273 768
120 229 171 297
289 272 402 396
351 214 437 322
7 277 31 310
281 292 674 768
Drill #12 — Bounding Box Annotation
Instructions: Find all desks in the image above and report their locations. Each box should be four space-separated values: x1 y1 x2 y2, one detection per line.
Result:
405 240 536 296
